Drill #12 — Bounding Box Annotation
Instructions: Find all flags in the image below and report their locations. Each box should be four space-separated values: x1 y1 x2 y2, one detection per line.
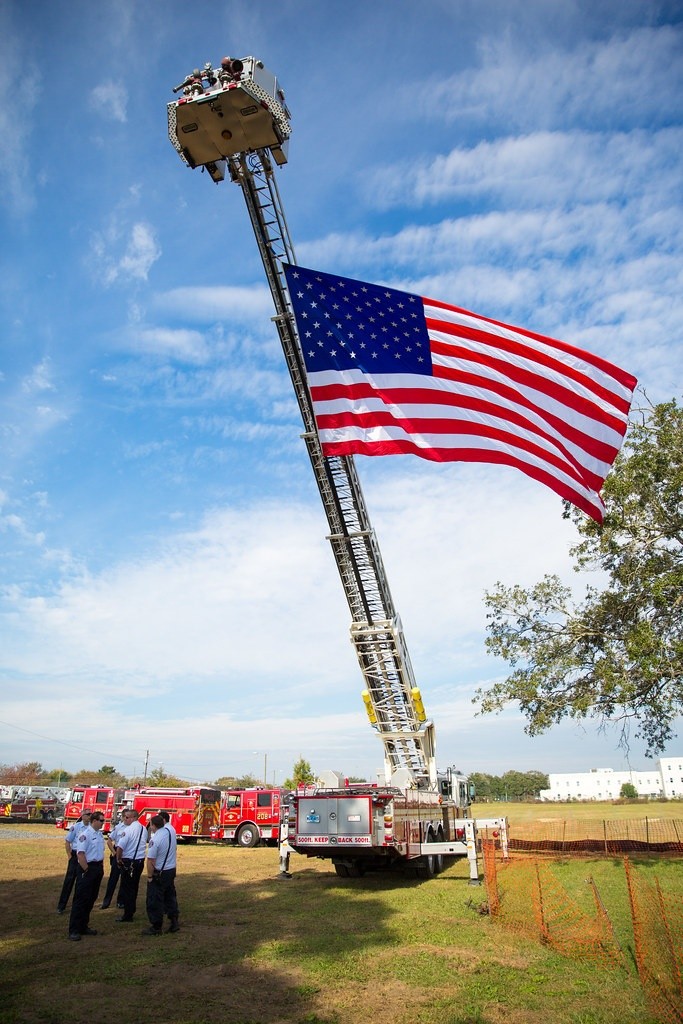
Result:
282 260 640 528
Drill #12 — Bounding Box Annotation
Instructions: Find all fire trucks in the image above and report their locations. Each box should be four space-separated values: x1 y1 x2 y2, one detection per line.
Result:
209 786 295 848
0 785 69 824
166 49 513 888
62 783 221 844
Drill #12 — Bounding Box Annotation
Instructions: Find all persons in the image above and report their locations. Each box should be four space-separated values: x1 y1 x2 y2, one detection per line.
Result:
57 808 182 941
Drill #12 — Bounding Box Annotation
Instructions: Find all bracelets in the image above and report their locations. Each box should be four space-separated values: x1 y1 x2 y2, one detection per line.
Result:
148 876 153 879
84 867 89 871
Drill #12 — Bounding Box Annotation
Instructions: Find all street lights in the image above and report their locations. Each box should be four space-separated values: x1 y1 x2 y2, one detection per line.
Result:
252 751 266 786
272 769 282 785
144 760 163 782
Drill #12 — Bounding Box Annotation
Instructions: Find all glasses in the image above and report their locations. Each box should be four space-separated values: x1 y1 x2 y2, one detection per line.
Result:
83 813 92 816
126 816 133 819
97 820 104 822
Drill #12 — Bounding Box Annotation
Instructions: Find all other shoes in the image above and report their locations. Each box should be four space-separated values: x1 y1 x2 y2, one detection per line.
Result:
118 903 124 909
100 905 109 910
143 927 162 935
69 932 82 941
58 909 64 915
165 922 179 933
116 917 133 922
78 927 97 936
